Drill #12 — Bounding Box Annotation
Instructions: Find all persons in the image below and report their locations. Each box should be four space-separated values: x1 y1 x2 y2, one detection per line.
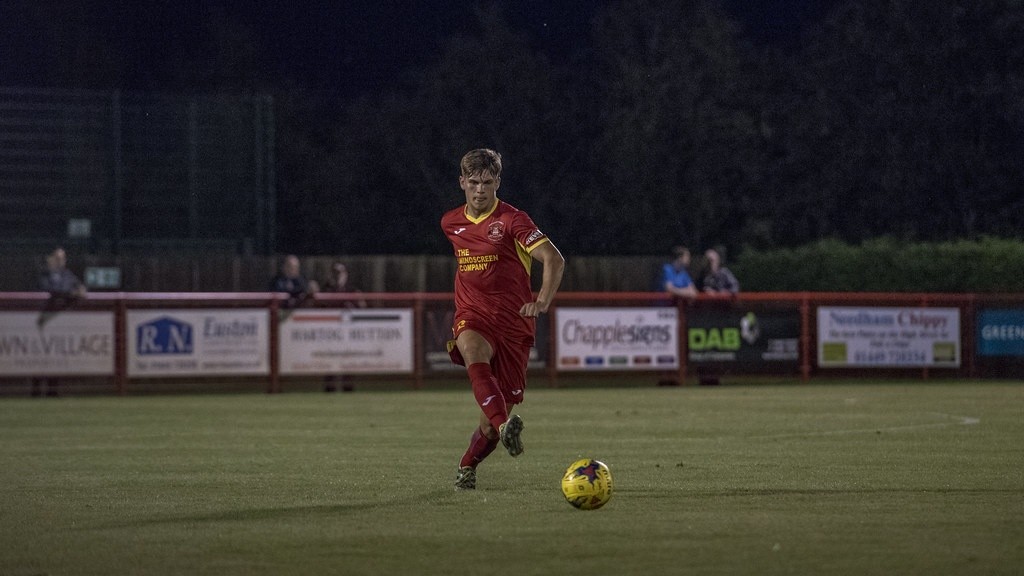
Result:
313 260 367 392
699 250 740 385
269 255 320 393
654 246 696 386
28 245 85 399
441 149 565 489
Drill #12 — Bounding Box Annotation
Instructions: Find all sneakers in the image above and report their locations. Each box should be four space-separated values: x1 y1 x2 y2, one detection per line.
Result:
499 414 524 457
457 454 476 490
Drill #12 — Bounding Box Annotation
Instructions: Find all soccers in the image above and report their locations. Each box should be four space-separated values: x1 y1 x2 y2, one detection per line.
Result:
559 457 616 511
